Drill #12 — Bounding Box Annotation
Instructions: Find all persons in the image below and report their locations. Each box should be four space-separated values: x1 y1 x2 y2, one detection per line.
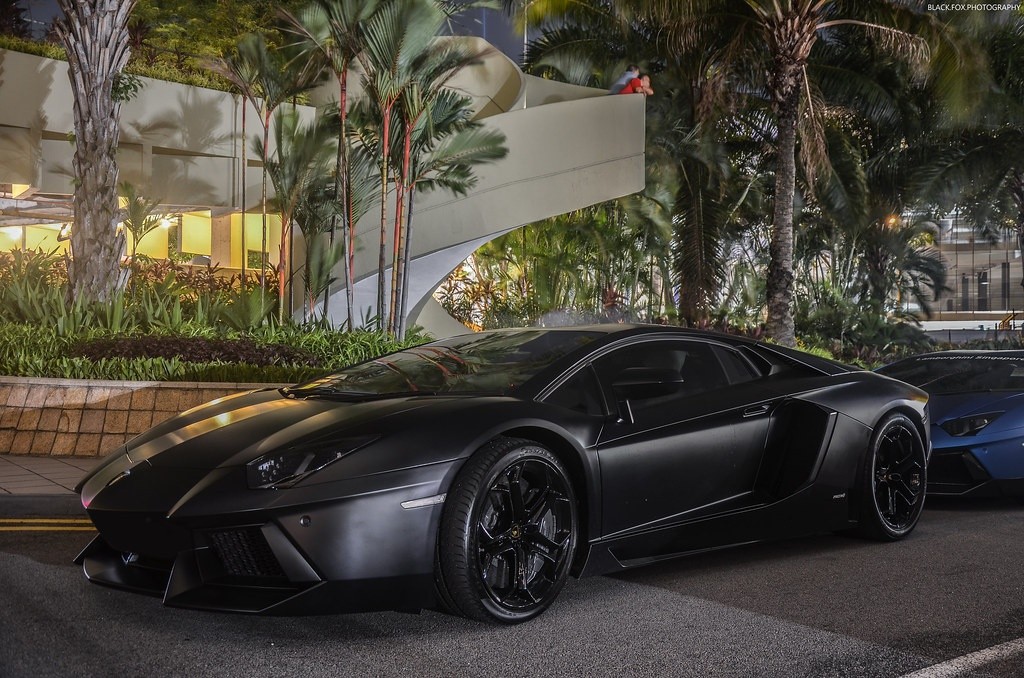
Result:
608 64 653 95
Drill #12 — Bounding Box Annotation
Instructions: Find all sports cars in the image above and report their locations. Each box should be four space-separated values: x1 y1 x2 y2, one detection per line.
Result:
871 349 1024 505
71 322 933 626
